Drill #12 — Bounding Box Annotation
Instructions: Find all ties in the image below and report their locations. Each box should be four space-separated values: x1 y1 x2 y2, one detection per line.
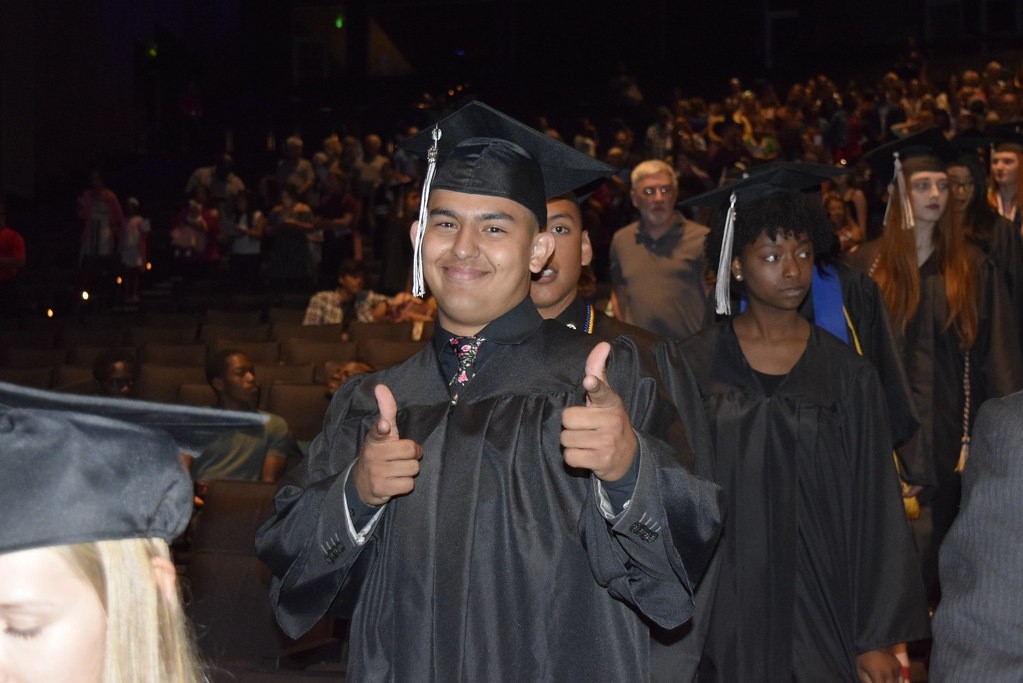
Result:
448 336 486 407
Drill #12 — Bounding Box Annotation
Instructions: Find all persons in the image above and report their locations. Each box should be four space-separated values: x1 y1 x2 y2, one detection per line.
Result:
1 380 271 683
0 33 1023 683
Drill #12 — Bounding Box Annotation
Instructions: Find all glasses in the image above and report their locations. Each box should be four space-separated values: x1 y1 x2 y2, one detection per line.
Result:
948 177 975 194
645 186 668 194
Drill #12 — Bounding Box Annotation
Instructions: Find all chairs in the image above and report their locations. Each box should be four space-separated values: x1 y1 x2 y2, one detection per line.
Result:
0 307 437 683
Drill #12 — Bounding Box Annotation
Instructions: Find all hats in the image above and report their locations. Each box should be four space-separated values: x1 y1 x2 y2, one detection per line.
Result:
404 100 615 297
676 165 831 316
949 129 984 173
0 381 272 552
989 132 1023 155
863 125 956 229
